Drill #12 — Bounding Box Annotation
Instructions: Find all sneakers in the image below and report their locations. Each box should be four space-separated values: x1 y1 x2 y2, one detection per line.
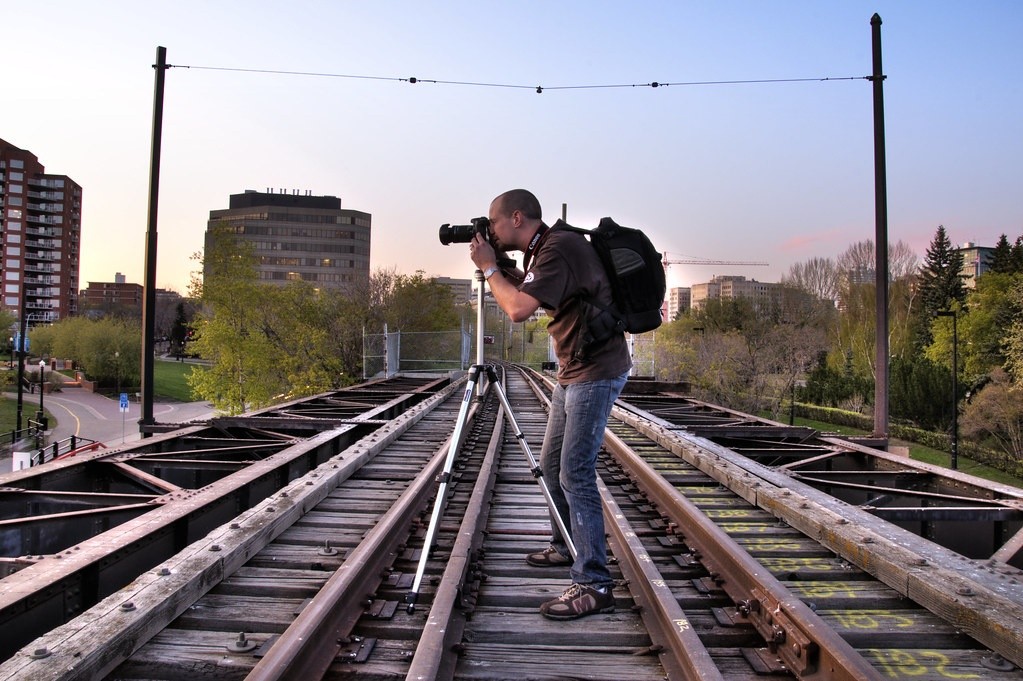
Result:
541 582 615 618
526 544 572 566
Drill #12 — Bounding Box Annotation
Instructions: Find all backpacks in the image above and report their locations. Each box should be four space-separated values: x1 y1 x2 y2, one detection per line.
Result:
533 217 667 334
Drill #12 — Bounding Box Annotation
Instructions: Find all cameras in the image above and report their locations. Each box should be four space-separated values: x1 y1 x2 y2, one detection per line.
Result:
440 217 491 246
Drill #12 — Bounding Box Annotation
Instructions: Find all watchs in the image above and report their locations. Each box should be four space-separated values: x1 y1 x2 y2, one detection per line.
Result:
484 265 500 280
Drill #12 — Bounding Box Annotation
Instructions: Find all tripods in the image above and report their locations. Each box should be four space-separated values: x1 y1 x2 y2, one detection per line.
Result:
405 271 580 614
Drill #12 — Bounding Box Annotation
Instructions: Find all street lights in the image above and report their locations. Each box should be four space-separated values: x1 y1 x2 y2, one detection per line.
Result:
38 360 45 414
10 337 14 369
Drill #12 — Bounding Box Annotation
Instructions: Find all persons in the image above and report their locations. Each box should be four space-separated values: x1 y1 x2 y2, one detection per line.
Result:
470 189 634 621
